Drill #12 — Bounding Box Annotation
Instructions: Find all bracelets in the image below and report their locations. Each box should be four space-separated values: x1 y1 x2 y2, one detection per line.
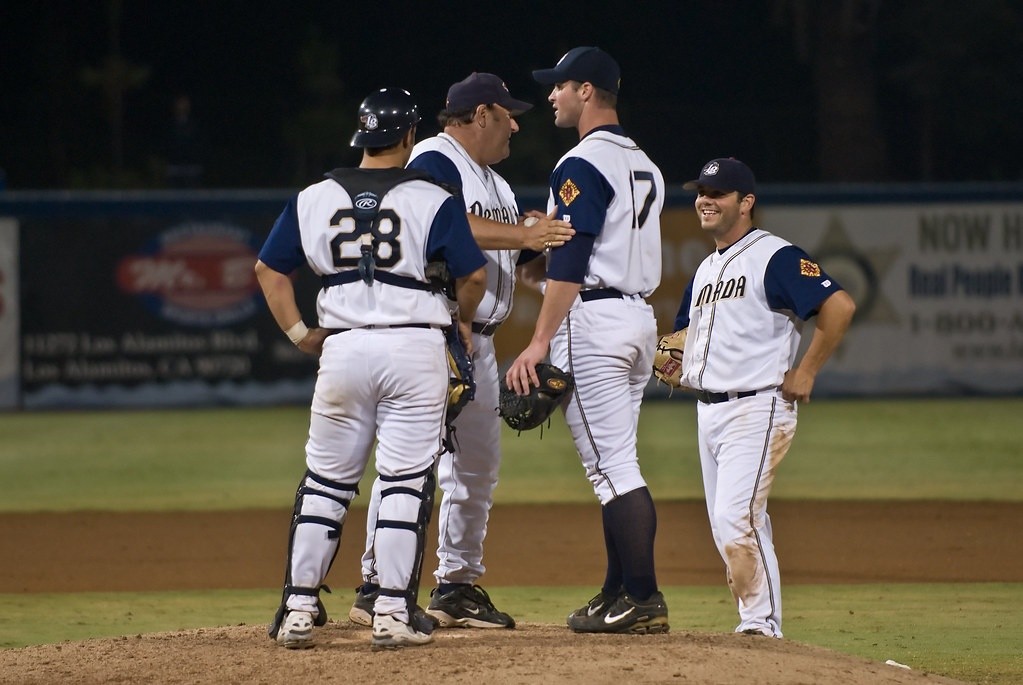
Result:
285 321 308 344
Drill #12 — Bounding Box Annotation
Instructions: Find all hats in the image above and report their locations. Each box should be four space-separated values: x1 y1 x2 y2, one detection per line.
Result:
447 72 533 117
532 47 620 94
684 157 756 194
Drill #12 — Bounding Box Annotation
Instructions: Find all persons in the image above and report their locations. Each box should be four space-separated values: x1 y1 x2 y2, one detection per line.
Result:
654 158 857 639
255 86 487 650
501 46 672 633
348 74 575 631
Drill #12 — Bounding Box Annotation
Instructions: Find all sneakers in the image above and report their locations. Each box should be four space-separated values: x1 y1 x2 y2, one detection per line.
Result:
566 593 618 624
370 612 433 651
568 591 671 634
348 585 440 629
424 584 515 628
274 611 315 648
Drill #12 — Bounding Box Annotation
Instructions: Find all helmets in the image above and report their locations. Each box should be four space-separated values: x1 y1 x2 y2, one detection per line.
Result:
350 87 421 148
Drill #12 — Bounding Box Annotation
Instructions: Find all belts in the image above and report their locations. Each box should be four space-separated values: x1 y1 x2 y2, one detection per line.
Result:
336 323 430 333
472 322 497 336
579 288 642 302
696 390 756 404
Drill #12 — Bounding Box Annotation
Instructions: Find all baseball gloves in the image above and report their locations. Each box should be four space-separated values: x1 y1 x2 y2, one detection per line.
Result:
494 363 574 441
652 326 689 399
438 317 478 457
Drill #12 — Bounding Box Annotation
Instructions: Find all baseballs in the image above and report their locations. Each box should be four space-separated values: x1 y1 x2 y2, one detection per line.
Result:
524 217 539 227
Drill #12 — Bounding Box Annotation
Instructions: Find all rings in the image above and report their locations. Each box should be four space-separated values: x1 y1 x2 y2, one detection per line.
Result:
544 242 551 247
